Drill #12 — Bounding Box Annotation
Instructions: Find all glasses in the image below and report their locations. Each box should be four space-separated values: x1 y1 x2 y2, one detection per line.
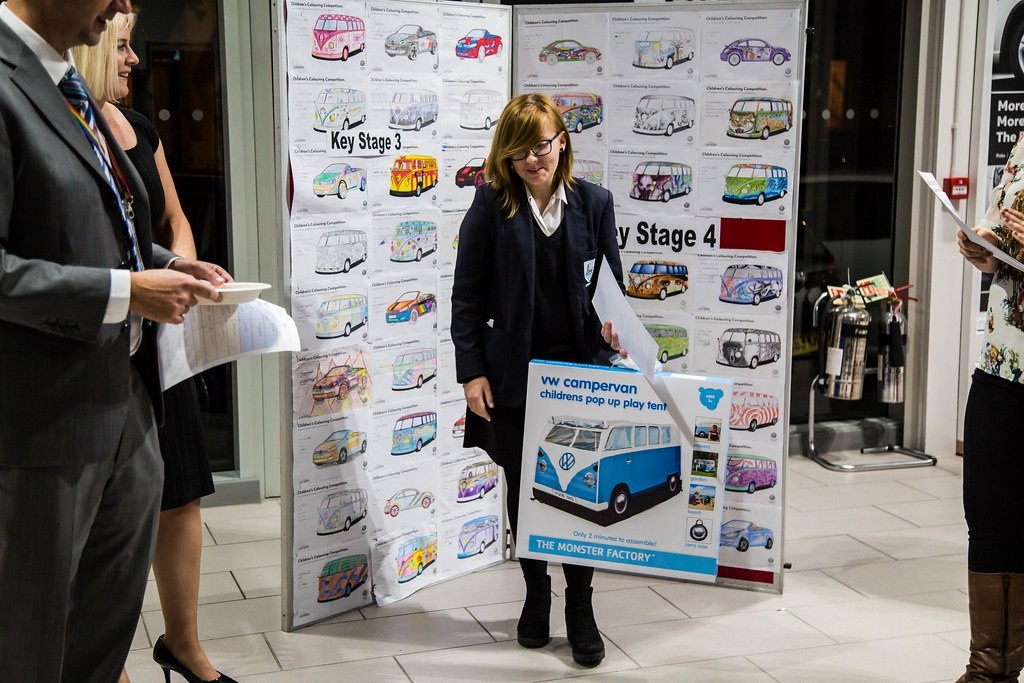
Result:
509 130 563 160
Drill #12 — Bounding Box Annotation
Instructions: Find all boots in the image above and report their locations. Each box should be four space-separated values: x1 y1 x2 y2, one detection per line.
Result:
955 568 1024 683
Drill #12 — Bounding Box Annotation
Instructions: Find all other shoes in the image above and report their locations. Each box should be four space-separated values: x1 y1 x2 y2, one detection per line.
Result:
517 575 551 648
565 588 604 661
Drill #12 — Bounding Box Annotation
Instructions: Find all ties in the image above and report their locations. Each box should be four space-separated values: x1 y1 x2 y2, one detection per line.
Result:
60 66 142 275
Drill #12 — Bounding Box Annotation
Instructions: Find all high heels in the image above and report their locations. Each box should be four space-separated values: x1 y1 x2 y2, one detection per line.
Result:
153 634 237 683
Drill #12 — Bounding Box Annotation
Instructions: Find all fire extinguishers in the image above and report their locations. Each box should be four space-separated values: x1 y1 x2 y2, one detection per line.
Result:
820 281 876 401
876 285 918 404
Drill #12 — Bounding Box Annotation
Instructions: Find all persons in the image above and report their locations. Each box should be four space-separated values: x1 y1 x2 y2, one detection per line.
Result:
0 0 231 682
68 0 240 682
963 131 1024 682
451 94 628 669
